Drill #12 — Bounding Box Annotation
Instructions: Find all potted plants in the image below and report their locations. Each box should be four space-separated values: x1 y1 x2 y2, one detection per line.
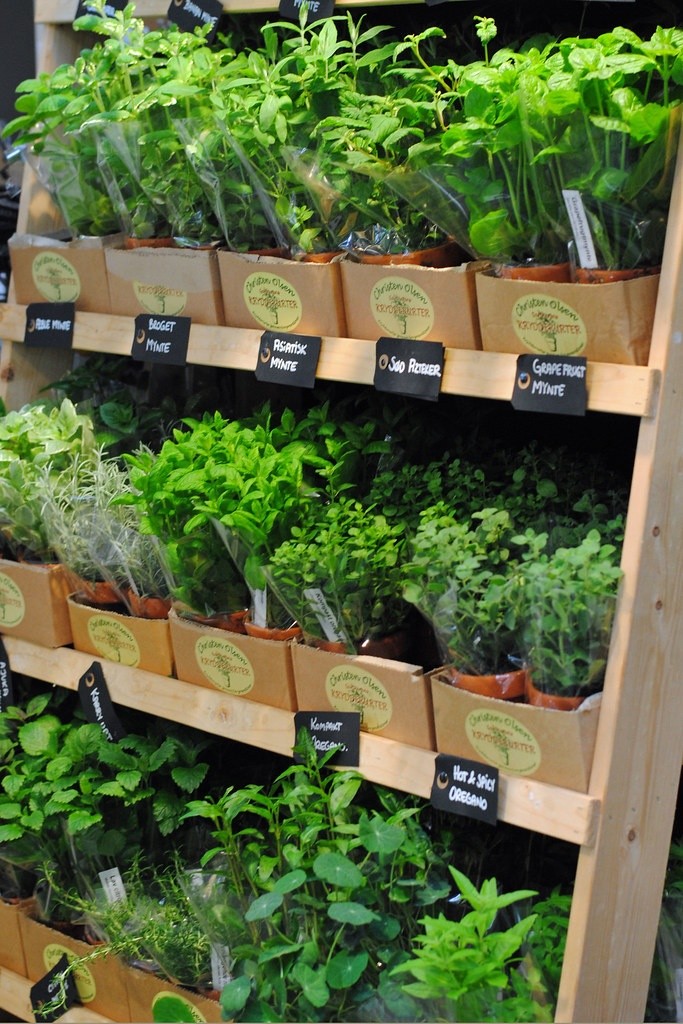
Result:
0 0 683 1024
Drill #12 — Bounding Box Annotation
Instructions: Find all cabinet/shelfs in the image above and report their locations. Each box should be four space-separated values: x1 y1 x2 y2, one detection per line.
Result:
0 0 683 1024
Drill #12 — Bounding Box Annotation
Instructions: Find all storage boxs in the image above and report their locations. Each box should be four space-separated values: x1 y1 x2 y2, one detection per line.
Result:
65 588 176 676
430 660 604 794
17 903 131 1024
475 264 661 368
0 555 73 647
336 251 491 353
215 245 349 339
102 237 228 328
8 229 124 314
0 896 37 978
285 629 455 752
124 960 235 1024
167 602 298 715
